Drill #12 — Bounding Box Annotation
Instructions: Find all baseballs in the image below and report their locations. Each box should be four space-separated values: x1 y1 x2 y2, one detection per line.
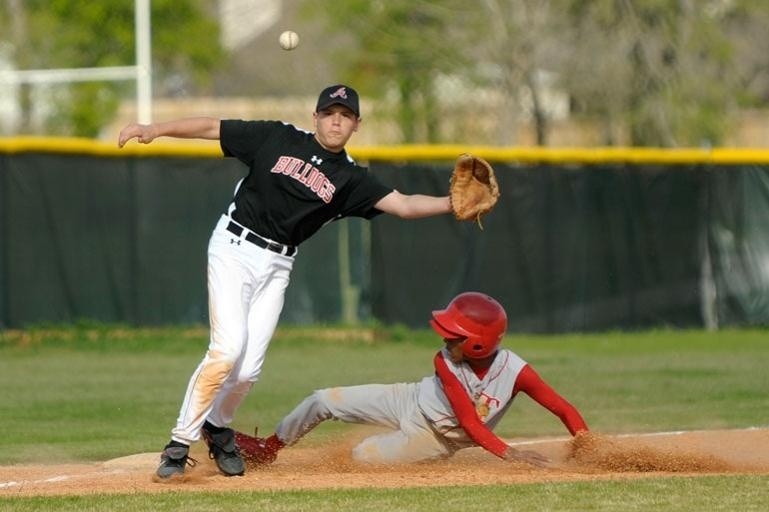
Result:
278 29 300 50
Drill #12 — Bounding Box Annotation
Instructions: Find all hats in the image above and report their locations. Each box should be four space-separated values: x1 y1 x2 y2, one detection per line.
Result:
317 85 359 116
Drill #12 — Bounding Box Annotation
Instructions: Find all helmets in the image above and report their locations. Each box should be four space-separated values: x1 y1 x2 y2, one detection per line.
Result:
429 290 508 358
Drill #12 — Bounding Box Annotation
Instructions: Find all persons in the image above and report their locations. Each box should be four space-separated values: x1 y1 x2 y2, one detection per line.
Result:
116 83 500 485
231 289 597 472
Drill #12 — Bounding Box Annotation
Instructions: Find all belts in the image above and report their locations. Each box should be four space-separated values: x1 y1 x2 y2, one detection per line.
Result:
227 222 295 257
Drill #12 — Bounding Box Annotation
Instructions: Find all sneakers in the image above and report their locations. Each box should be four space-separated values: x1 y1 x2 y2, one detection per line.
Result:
201 425 243 477
153 445 189 482
233 431 277 471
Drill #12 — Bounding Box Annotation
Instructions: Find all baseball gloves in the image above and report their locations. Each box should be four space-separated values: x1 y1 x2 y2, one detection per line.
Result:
449 153 501 222
